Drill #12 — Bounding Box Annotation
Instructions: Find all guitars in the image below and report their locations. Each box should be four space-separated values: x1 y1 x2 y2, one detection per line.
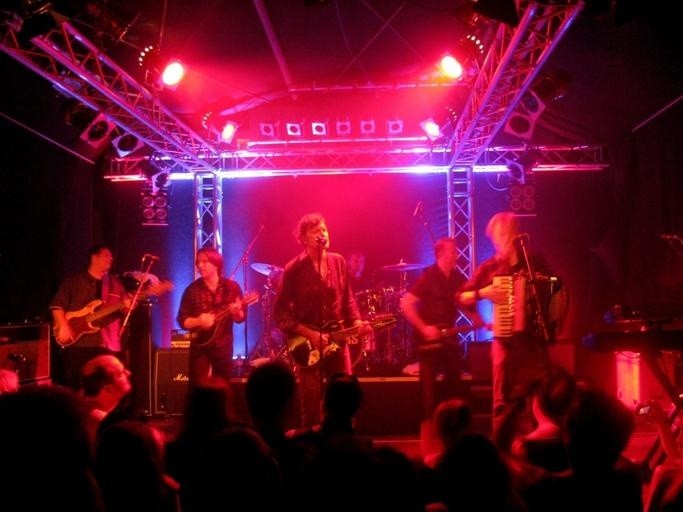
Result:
410 322 491 352
286 317 398 369
52 281 173 348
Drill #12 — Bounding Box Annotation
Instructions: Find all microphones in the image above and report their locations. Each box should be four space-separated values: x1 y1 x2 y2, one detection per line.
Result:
413 202 422 217
314 236 326 245
144 253 159 260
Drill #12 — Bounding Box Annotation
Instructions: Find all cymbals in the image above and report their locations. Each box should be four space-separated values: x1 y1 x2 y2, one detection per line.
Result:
379 263 429 271
250 263 283 276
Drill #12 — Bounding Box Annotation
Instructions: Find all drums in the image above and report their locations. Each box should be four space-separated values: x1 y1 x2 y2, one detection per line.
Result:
353 286 399 322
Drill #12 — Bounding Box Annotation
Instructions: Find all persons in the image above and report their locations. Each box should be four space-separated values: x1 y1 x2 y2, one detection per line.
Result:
270 211 364 432
48 240 137 401
1 352 682 512
454 209 566 417
397 234 484 435
172 246 248 396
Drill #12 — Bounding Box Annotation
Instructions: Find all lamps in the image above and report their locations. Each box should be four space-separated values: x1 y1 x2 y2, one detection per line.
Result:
128 38 184 89
67 98 143 159
438 32 487 79
415 100 463 139
136 166 173 229
502 67 574 221
200 112 240 145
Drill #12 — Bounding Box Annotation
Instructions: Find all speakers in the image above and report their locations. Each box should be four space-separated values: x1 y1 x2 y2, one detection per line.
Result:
231 380 304 428
356 377 419 435
153 348 191 413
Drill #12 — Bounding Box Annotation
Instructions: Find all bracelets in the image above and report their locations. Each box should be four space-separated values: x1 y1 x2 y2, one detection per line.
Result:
473 289 483 302
